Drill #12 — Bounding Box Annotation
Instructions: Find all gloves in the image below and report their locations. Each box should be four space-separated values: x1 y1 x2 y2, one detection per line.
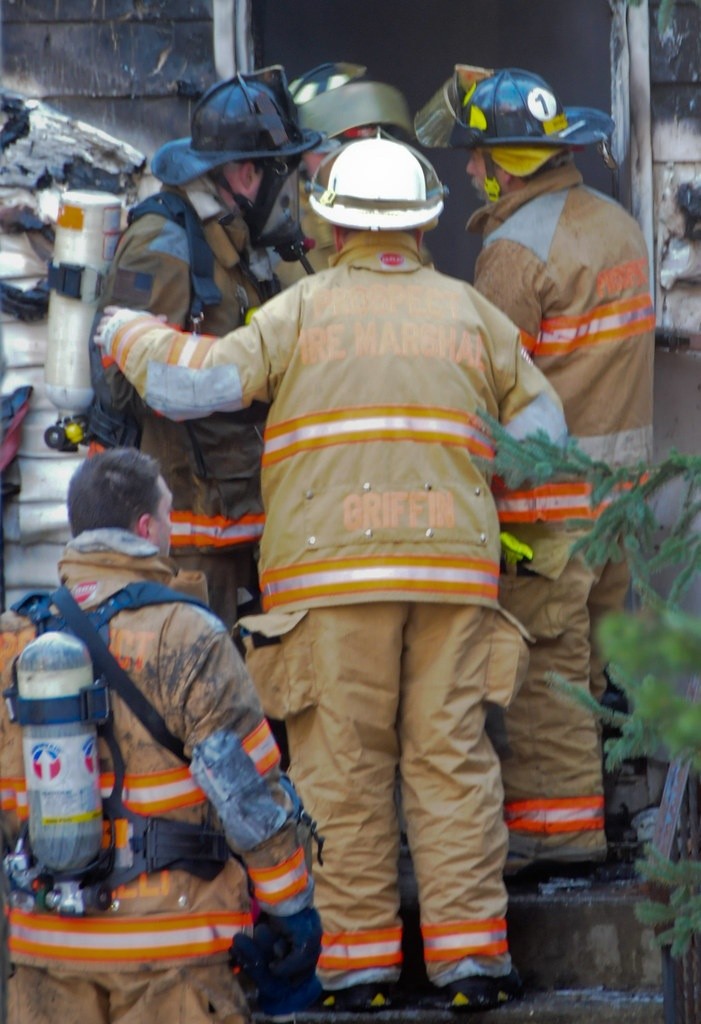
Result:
226 906 323 1002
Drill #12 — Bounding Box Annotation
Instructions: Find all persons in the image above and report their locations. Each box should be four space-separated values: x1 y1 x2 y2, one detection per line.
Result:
90 60 437 663
415 61 654 896
94 140 568 1013
0 449 322 1024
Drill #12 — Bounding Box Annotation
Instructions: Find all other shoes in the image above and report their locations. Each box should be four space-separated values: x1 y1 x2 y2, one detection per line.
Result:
502 860 593 894
448 974 523 1008
320 983 392 1012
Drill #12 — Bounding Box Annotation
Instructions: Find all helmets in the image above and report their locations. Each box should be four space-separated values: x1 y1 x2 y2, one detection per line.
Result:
414 67 615 146
309 137 444 231
150 64 322 187
288 63 413 139
